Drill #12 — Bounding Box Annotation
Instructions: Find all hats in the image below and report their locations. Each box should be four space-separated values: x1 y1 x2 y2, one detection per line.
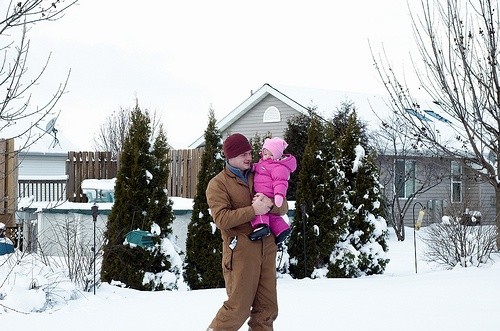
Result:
223 133 252 159
261 137 288 161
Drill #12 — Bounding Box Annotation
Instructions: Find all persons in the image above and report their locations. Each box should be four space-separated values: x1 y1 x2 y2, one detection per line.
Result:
205 134 278 331
248 137 297 245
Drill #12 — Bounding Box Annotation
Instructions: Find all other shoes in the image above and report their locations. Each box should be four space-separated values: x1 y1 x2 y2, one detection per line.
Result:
275 228 292 245
248 225 270 240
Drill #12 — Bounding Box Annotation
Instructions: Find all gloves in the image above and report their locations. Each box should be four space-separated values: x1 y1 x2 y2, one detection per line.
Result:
275 195 283 207
